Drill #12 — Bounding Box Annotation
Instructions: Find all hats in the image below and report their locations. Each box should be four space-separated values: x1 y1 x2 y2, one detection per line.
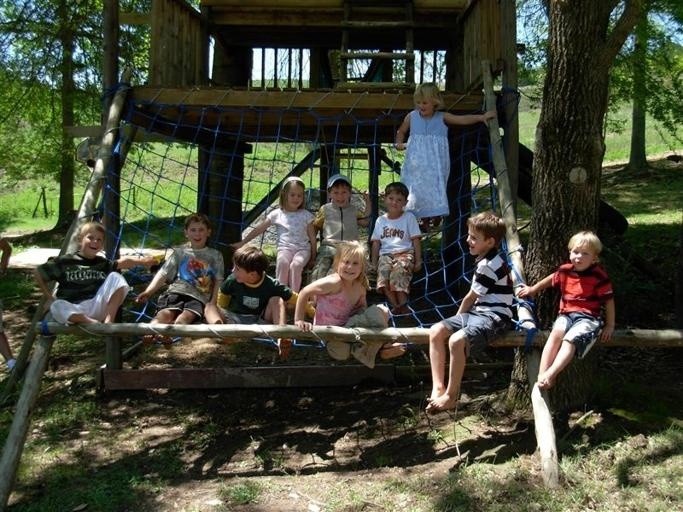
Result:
326 175 351 193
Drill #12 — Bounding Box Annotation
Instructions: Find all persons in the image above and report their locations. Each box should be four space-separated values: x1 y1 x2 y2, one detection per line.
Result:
32 221 161 326
293 239 406 362
308 174 366 287
516 229 617 389
424 210 514 412
0 235 18 370
216 244 315 360
395 81 496 232
227 175 318 312
370 181 423 314
137 212 224 346
